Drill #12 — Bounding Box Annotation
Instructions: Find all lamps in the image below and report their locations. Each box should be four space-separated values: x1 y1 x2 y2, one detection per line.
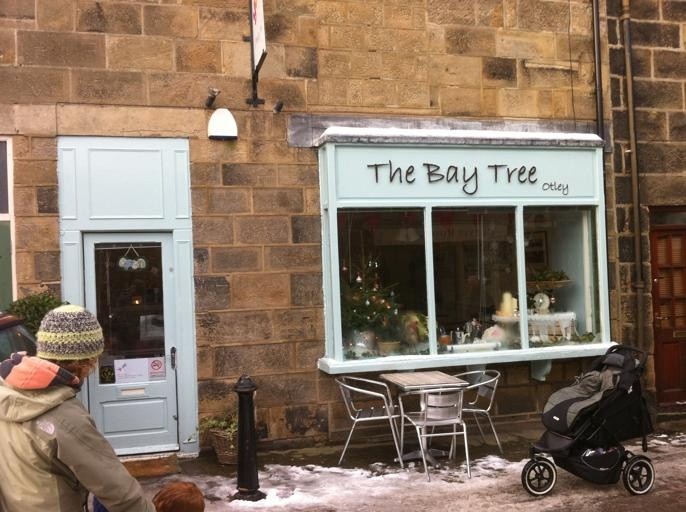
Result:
207 107 239 141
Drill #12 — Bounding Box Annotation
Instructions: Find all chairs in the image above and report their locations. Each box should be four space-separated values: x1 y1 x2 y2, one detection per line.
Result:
334 368 504 482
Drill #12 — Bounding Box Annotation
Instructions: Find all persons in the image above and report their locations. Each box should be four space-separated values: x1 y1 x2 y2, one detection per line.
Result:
0 289 68 372
0 304 158 511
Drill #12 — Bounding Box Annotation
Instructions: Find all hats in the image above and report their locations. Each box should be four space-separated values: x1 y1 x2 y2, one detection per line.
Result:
36 304 104 362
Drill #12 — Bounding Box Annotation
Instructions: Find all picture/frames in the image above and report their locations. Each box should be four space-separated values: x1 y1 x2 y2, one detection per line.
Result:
525 231 549 272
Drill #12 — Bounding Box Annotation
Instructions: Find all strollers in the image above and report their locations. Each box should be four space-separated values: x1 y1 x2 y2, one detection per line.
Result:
521 345 656 496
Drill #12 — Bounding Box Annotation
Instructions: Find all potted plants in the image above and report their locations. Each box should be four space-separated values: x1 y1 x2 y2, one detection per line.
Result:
375 324 401 356
200 400 240 465
